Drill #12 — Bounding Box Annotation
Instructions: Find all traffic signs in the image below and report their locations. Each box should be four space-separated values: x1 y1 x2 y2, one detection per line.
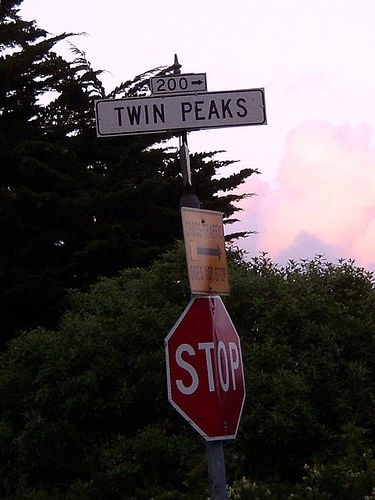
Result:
95 88 268 136
181 207 231 298
150 72 208 97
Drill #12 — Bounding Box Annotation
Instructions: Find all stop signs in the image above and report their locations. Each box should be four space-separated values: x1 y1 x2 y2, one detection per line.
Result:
164 293 246 440
